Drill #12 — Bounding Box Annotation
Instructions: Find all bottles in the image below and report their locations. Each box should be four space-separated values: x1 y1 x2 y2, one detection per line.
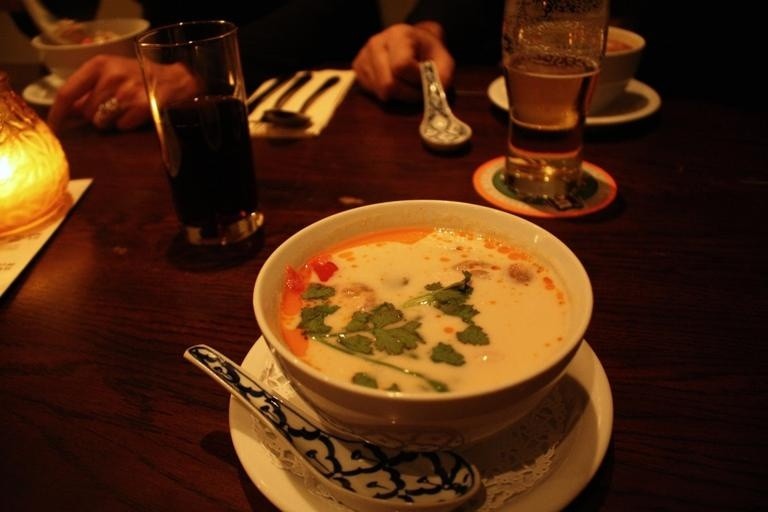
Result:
0 75 71 238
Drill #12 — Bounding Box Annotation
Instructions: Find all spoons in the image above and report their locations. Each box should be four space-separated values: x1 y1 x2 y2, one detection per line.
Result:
417 57 473 148
26 2 81 46
183 343 480 511
265 76 340 130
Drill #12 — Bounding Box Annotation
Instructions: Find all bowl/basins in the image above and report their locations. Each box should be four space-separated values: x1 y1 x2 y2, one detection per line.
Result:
519 24 646 113
253 199 597 451
33 19 148 76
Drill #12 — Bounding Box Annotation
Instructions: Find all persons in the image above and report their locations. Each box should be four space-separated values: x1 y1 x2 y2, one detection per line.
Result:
352 1 453 111
45 1 210 134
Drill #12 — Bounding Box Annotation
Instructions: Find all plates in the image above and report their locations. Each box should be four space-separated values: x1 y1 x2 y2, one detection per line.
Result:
25 76 66 105
228 332 616 509
489 76 660 130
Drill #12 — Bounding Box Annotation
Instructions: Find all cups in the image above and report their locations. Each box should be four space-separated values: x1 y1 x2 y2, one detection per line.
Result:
499 1 608 200
136 20 267 244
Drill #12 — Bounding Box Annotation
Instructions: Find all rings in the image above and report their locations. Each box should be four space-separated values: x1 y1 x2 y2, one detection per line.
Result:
94 97 119 123
